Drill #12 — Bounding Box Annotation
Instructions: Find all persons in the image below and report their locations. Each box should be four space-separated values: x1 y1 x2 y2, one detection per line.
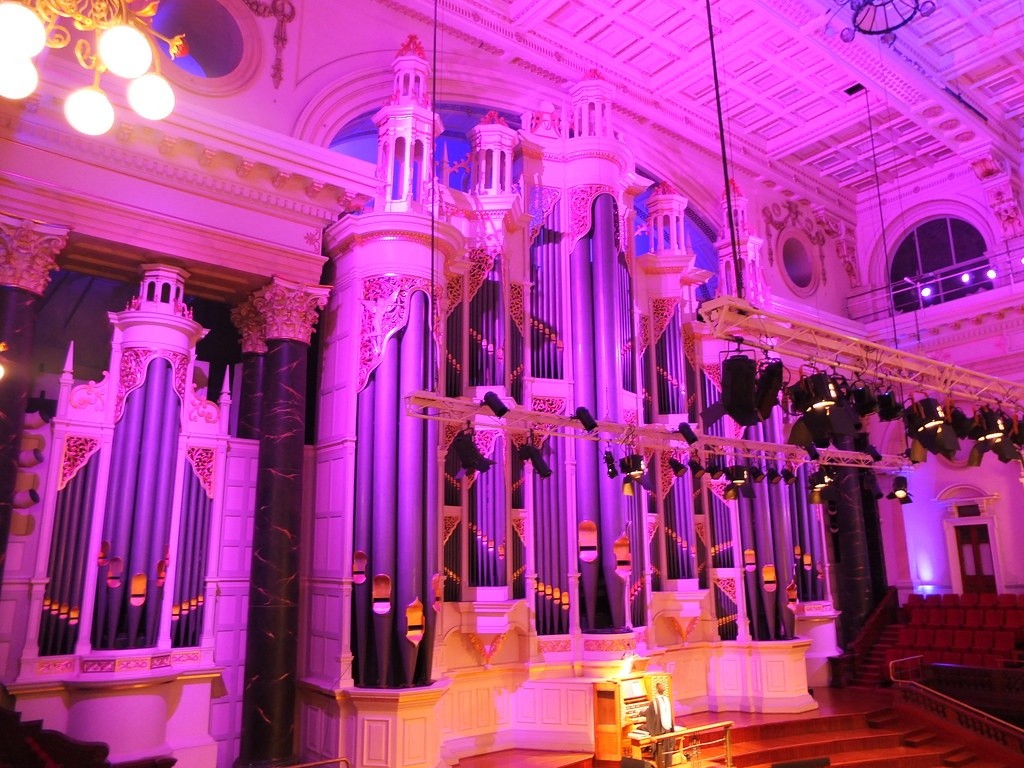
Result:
637 683 674 767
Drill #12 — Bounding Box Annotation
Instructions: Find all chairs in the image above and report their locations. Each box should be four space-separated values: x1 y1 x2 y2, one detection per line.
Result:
884 592 1024 689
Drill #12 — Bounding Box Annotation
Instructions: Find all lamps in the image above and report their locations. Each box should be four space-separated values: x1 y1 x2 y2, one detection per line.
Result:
0 0 191 137
824 0 937 49
452 338 1024 502
